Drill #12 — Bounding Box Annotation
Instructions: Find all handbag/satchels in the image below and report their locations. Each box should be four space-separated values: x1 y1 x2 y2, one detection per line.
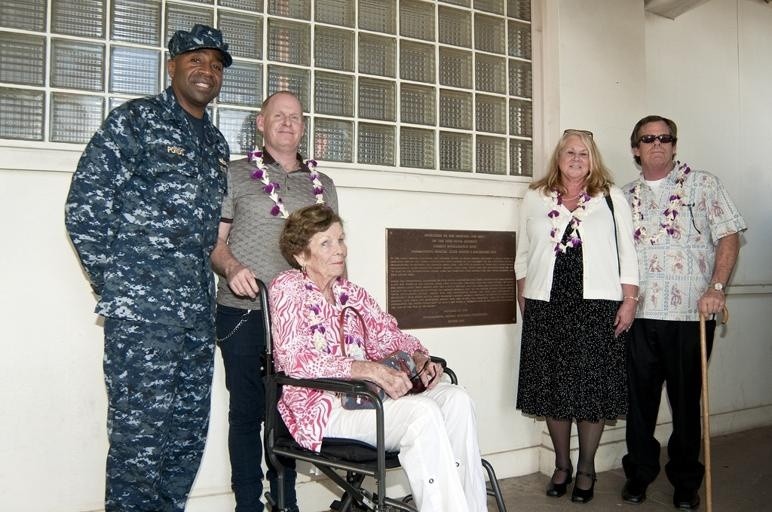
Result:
341 350 417 409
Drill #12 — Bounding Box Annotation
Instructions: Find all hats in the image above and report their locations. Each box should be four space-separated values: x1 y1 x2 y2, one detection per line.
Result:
168 24 232 68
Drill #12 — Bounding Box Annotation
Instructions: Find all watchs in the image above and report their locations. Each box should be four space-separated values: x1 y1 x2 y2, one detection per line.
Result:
709 282 728 291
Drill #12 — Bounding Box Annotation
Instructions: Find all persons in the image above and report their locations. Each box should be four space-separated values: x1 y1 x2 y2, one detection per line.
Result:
217 89 348 512
513 128 638 503
265 202 490 512
617 115 747 509
65 24 233 512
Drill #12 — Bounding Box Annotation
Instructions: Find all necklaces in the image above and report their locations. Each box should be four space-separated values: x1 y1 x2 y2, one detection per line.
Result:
561 192 585 203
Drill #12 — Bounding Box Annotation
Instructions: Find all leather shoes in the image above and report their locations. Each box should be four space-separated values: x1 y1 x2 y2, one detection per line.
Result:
571 471 596 504
546 459 573 497
673 488 700 511
621 481 648 503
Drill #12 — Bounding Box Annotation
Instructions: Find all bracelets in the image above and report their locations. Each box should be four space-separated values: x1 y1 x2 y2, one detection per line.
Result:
623 296 639 302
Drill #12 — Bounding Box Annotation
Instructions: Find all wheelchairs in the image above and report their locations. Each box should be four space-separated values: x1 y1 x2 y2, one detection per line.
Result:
248 277 507 511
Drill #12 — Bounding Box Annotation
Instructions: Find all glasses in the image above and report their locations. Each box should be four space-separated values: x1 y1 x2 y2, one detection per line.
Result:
564 129 593 140
636 134 675 146
410 361 436 394
